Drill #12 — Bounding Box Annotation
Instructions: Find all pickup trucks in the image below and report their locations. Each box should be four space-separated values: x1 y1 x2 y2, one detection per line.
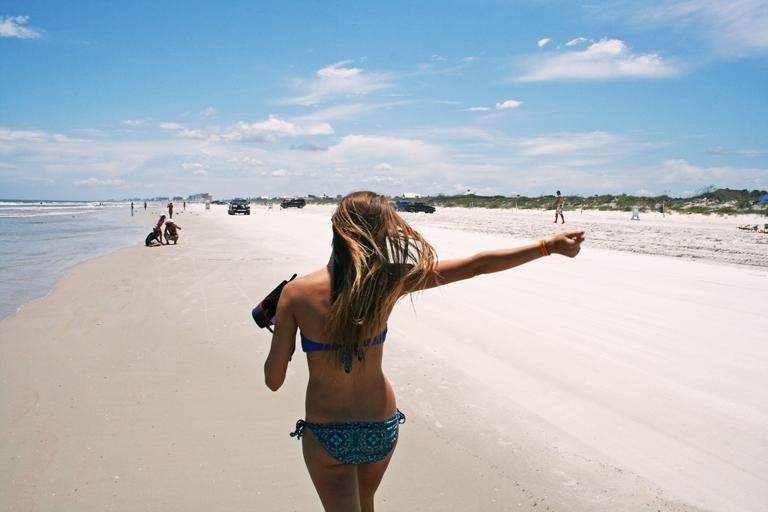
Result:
404 202 436 214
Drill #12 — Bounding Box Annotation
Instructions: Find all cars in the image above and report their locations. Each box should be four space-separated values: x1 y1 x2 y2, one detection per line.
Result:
387 200 413 212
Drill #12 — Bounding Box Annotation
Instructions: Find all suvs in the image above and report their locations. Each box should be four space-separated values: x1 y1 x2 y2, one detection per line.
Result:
280 197 306 209
227 198 251 216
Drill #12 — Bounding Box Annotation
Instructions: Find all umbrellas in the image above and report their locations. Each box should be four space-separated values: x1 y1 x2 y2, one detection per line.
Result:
752 194 768 203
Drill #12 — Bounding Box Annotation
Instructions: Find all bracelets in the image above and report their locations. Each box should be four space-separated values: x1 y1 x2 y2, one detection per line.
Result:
538 238 551 257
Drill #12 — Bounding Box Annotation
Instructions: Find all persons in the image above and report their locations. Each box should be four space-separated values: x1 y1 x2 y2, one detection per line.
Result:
163 219 181 245
261 190 587 512
144 229 166 248
167 203 173 218
553 189 566 226
153 212 166 245
